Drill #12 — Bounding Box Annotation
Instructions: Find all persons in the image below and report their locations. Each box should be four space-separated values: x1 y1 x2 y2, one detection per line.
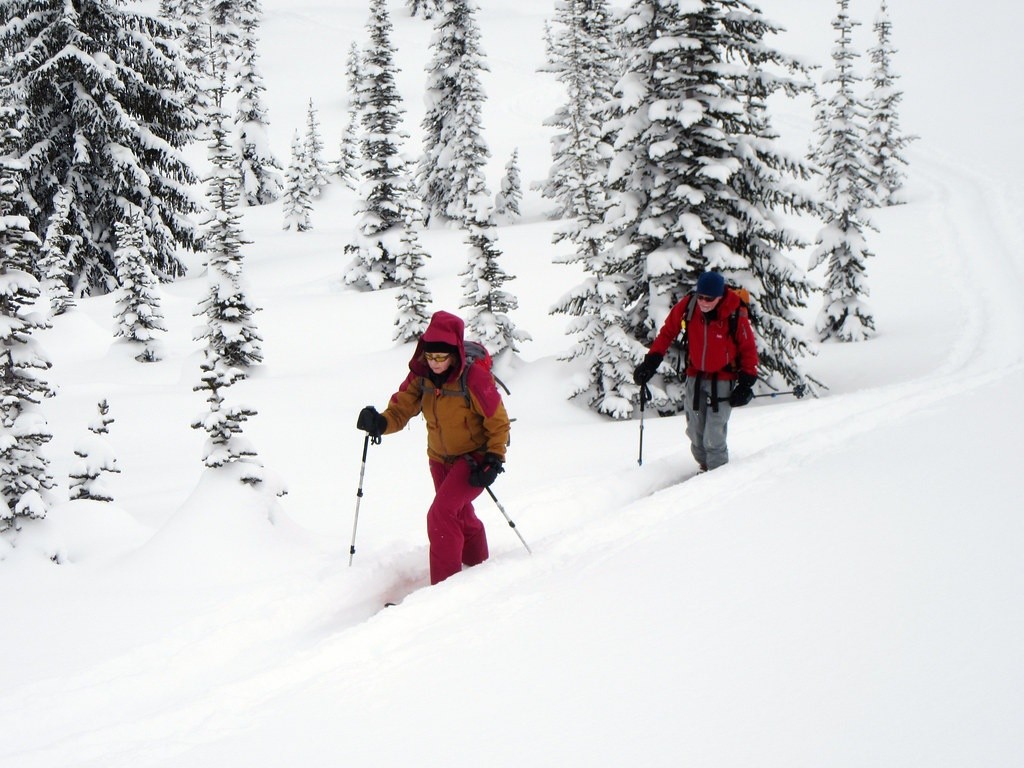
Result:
357 311 511 584
633 271 758 475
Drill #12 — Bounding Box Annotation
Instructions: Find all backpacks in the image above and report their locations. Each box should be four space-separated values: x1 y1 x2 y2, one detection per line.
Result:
418 340 512 446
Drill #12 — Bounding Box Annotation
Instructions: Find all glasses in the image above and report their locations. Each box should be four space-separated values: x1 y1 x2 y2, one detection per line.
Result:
695 295 718 302
423 353 452 362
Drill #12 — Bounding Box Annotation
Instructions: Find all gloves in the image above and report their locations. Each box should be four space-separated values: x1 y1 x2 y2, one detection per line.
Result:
633 352 663 386
729 372 757 407
356 406 387 437
469 453 505 487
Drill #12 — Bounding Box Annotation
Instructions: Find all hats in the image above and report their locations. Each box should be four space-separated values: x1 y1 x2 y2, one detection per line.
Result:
422 312 459 352
696 272 725 295
